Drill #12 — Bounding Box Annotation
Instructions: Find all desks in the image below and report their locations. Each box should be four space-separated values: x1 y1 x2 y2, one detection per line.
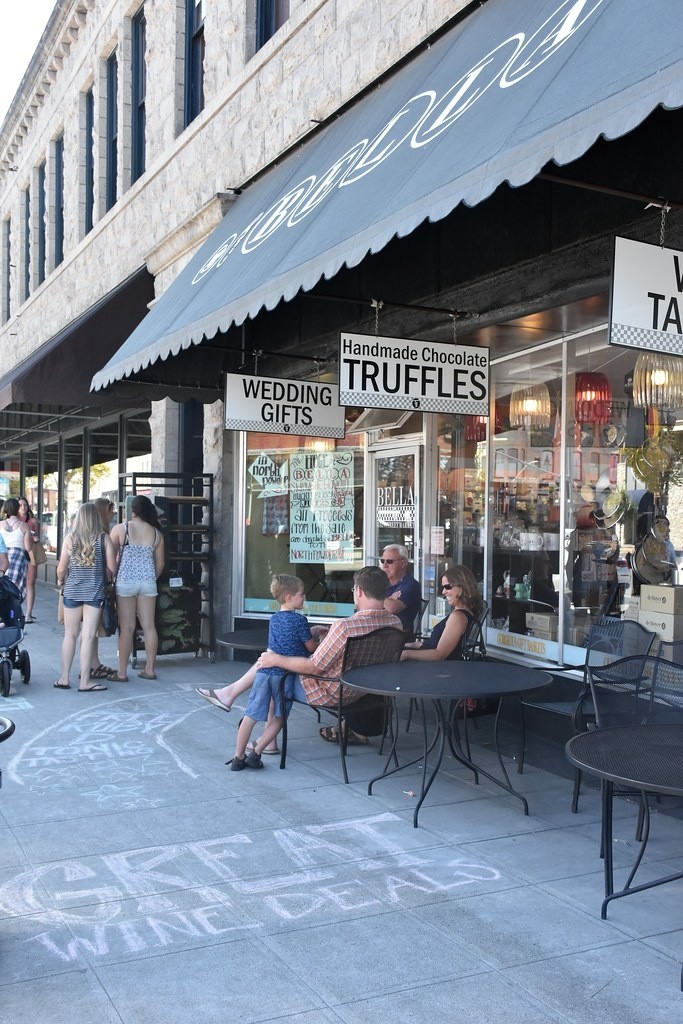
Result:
217 629 323 730
340 661 553 829
565 722 683 993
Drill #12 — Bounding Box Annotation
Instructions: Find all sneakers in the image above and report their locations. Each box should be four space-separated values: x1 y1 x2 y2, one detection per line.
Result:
230 753 247 771
244 749 263 768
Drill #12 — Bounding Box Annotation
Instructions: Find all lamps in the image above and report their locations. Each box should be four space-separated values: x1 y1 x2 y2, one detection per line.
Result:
465 400 503 442
633 352 683 409
304 436 335 453
574 337 612 424
510 355 550 432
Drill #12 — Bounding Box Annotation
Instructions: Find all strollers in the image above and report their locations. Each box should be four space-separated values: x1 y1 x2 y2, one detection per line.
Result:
0 575 31 697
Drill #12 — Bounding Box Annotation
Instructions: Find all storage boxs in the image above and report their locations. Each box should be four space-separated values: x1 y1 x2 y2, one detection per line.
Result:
524 613 591 647
621 584 683 681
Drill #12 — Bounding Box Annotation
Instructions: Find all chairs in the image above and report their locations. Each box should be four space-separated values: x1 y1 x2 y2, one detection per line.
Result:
519 621 655 814
586 654 683 859
279 598 489 786
571 640 683 841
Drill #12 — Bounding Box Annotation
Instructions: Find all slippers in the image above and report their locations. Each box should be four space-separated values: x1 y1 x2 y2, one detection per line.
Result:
53 681 70 689
25 618 33 623
107 673 127 682
195 688 230 712
244 740 278 754
77 684 106 691
78 668 106 679
319 726 368 744
137 671 155 679
96 664 117 673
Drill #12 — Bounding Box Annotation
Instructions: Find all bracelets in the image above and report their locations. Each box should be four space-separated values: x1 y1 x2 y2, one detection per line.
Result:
0 570 5 574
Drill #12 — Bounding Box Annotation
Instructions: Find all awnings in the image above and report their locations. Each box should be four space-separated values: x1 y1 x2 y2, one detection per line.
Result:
87 1 683 395
0 261 154 454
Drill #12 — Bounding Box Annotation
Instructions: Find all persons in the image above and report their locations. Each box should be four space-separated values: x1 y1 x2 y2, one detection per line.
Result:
230 573 319 769
108 495 165 682
0 496 40 627
53 498 118 691
320 564 482 746
194 565 403 757
309 544 421 644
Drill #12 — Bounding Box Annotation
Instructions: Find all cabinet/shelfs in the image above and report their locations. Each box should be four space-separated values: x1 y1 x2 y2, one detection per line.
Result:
117 472 214 669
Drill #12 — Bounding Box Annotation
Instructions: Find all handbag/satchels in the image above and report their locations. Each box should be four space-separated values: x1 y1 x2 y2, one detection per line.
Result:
449 656 500 717
101 597 116 636
58 583 66 625
28 541 47 565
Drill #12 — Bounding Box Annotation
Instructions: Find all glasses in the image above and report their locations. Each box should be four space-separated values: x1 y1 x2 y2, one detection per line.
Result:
441 583 461 591
380 559 402 564
351 588 358 592
